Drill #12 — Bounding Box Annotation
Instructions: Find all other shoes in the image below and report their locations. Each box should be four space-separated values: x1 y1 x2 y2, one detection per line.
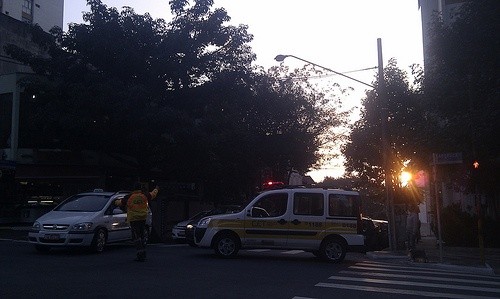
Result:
135 257 145 262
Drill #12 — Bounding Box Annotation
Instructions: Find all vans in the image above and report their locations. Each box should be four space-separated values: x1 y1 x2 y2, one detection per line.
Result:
194 188 365 263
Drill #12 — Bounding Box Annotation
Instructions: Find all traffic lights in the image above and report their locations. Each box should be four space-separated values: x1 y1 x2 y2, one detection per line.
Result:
472 160 480 169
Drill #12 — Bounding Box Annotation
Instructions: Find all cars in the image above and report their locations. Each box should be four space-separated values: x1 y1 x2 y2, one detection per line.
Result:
29 188 154 254
171 211 211 240
185 204 241 248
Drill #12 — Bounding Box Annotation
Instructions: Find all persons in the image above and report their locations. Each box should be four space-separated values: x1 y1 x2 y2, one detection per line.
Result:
406 207 421 253
114 181 160 263
